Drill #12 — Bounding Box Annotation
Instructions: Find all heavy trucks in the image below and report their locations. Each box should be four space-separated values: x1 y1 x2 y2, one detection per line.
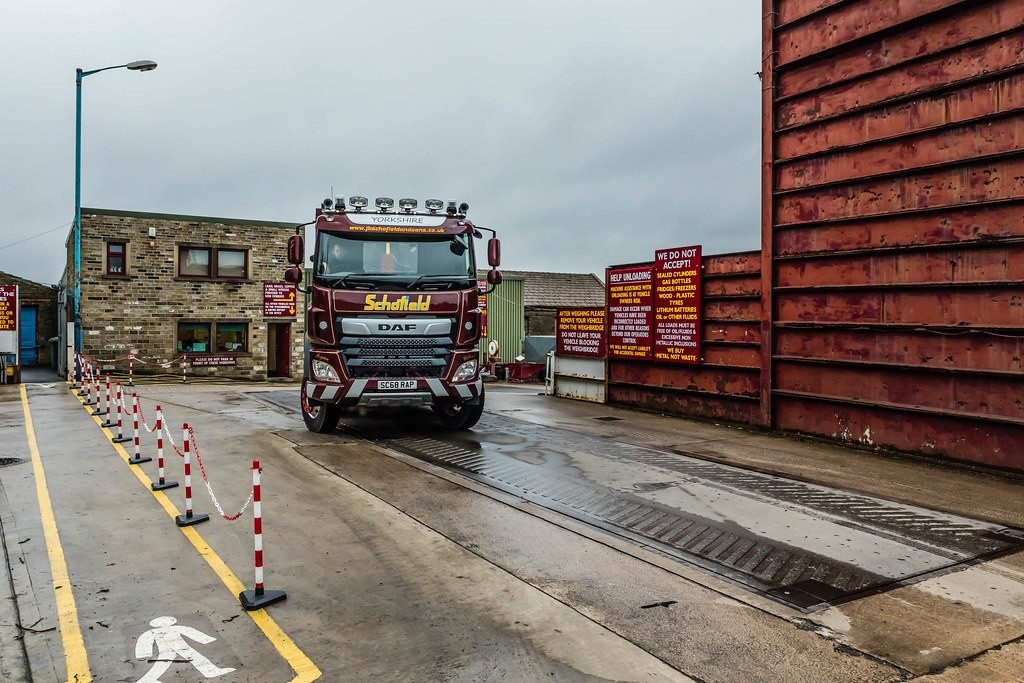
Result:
285 195 502 433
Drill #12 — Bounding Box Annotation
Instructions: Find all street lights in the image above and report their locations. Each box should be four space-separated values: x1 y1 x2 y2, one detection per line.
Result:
74 60 158 385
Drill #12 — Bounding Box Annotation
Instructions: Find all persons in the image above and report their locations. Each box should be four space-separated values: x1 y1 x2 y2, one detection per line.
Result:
328 244 365 273
